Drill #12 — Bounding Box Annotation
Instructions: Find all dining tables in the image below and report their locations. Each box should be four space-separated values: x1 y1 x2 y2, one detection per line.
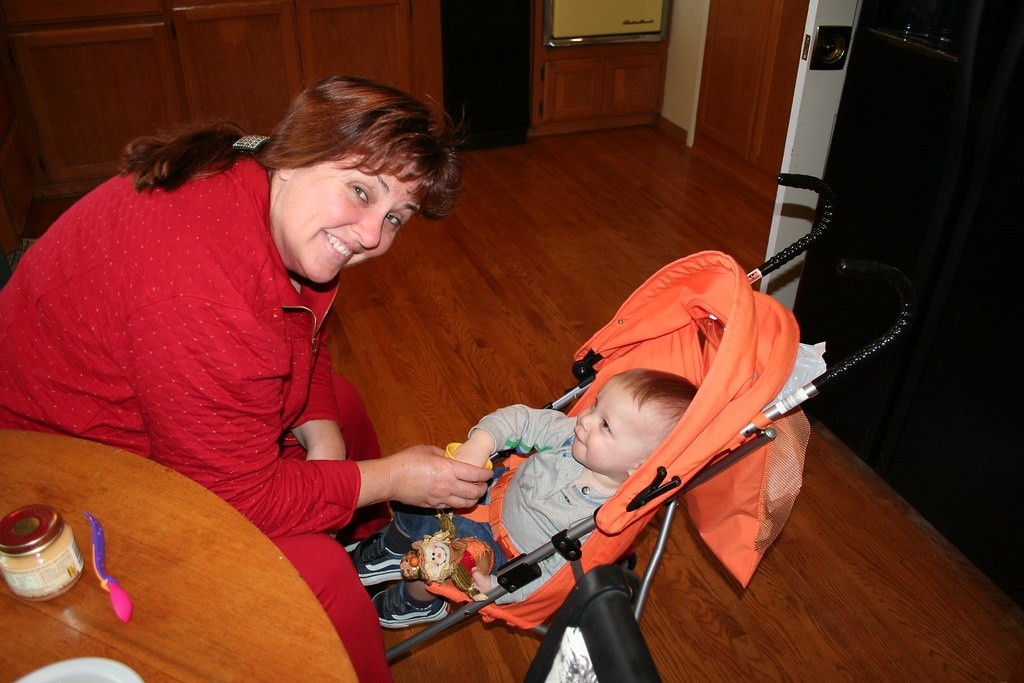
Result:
0 431 360 683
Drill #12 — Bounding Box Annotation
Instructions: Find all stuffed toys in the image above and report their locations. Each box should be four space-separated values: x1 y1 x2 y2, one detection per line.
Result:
400 510 496 602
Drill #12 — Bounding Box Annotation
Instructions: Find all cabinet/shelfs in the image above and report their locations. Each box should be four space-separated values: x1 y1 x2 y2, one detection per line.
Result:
0 0 186 200
0 2 39 254
527 41 673 143
691 0 812 204
171 0 444 151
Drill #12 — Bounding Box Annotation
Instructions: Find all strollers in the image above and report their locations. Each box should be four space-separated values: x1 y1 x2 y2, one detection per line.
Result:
330 173 918 665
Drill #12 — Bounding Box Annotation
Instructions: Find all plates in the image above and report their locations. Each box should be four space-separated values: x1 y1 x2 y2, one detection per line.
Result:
14 656 145 683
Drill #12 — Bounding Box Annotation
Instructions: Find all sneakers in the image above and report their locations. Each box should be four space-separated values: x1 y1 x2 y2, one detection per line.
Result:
371 580 450 629
343 525 405 586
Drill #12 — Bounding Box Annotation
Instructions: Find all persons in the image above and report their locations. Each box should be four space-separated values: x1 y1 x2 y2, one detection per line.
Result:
1 73 494 683
343 367 699 629
